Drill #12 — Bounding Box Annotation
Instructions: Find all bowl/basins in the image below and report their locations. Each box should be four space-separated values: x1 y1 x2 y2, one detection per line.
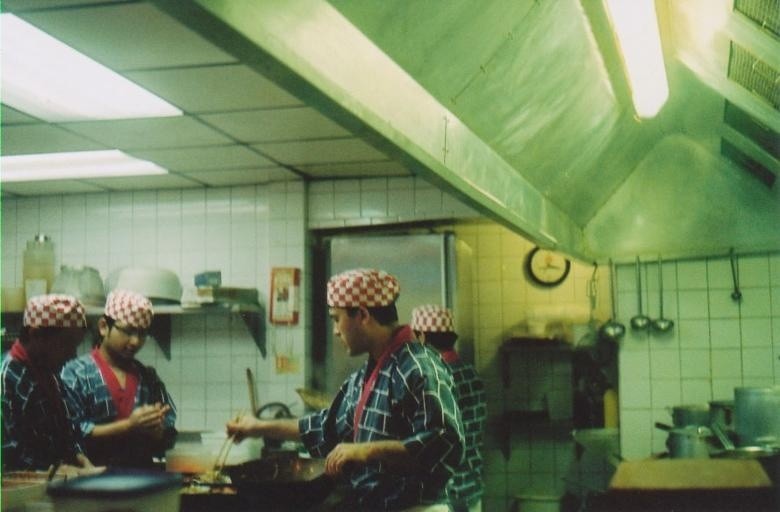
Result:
50 264 107 307
109 265 184 309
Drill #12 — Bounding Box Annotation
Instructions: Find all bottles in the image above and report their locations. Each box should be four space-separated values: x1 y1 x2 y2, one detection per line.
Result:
20 235 55 312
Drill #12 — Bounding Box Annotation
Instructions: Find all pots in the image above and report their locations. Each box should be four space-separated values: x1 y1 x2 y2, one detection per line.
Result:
672 386 780 457
219 454 341 500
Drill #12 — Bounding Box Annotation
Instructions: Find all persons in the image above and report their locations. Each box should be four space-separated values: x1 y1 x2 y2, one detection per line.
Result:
58 286 177 468
0 293 95 469
224 267 465 512
410 304 489 511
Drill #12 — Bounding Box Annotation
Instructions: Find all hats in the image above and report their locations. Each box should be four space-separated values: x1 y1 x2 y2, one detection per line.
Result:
325 268 401 308
103 288 153 330
23 293 87 328
409 304 455 335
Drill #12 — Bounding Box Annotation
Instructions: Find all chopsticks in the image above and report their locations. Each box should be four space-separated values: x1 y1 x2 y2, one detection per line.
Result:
209 409 245 487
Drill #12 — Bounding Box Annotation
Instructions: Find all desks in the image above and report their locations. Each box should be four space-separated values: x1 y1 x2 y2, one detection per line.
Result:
606 458 775 512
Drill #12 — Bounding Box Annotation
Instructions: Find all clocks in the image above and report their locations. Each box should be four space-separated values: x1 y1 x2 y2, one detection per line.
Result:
527 246 570 286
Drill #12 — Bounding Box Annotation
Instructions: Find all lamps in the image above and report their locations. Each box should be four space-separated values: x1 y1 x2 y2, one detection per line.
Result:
603 1 670 120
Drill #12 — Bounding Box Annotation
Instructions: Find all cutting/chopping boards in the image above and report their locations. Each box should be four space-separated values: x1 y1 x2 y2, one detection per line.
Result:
606 459 776 490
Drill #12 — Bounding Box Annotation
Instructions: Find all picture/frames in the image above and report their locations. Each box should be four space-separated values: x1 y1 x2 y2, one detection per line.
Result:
269 267 299 326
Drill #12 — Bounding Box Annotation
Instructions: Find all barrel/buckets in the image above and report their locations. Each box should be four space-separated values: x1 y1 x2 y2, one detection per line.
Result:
513 491 563 511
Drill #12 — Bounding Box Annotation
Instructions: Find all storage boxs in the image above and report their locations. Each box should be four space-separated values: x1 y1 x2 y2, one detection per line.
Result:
515 487 563 512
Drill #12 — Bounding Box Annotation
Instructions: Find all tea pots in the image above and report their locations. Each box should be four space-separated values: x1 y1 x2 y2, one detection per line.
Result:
654 420 715 458
